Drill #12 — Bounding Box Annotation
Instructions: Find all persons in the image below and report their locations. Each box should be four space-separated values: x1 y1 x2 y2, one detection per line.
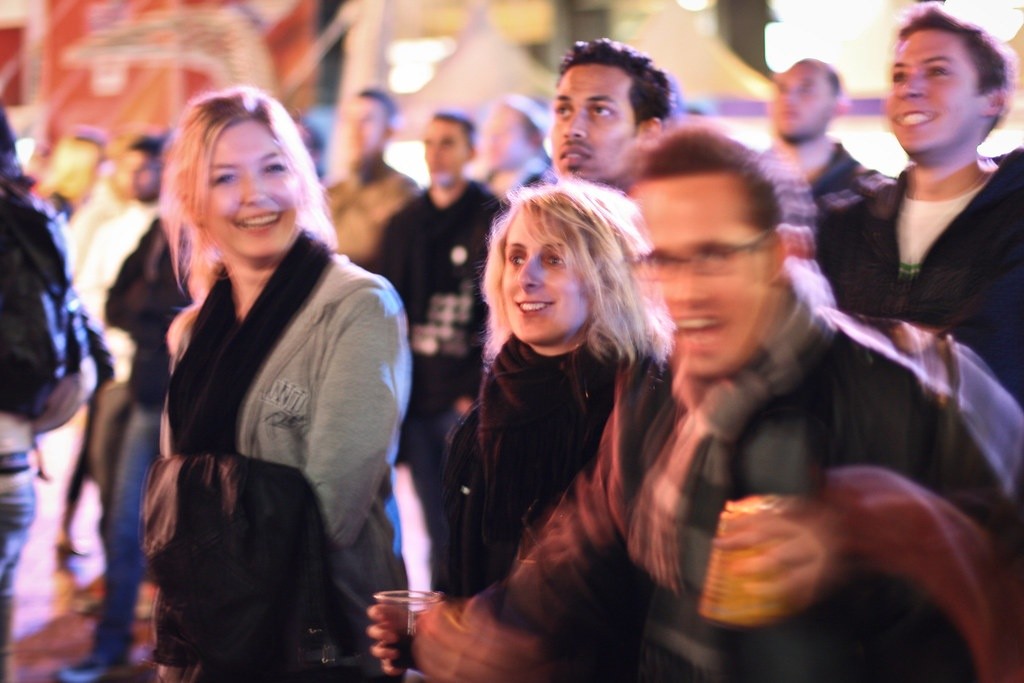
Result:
0 87 419 683
366 6 1024 683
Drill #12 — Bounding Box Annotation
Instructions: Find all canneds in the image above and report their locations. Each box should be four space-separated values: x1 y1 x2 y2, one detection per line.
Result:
700 499 783 625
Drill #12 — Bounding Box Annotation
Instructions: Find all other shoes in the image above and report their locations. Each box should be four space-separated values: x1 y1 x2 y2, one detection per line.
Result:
58 661 106 683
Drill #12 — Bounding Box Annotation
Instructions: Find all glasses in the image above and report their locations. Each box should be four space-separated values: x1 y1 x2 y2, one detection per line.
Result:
625 228 773 269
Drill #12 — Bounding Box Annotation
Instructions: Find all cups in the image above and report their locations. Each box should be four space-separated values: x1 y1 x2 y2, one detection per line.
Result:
699 495 790 629
374 591 442 677
